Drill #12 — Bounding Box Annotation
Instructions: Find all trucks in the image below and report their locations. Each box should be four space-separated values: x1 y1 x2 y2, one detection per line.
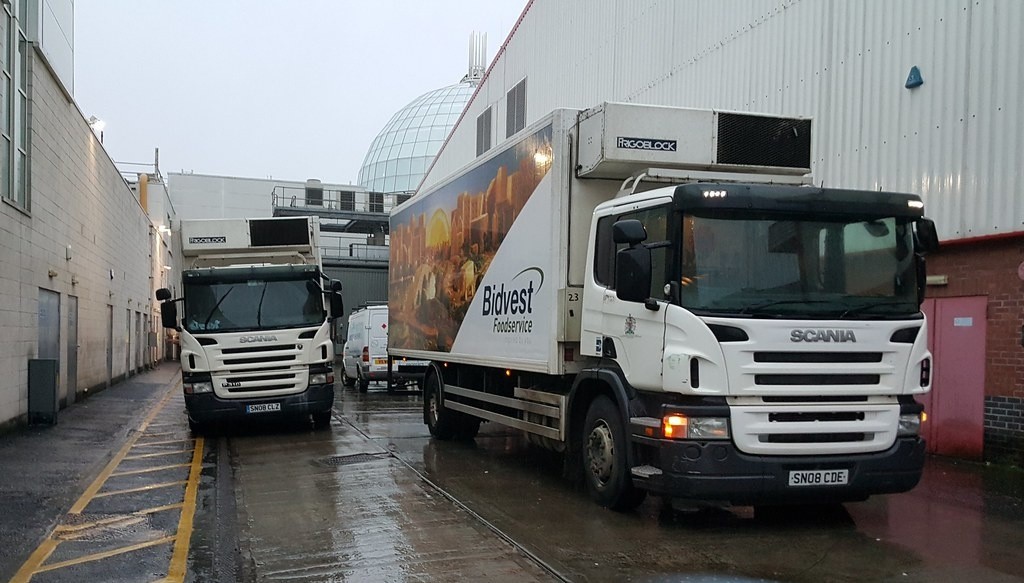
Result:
155 216 345 433
388 100 938 510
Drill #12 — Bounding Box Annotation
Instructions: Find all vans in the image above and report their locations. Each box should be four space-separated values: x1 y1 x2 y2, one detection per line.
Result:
341 300 430 391
